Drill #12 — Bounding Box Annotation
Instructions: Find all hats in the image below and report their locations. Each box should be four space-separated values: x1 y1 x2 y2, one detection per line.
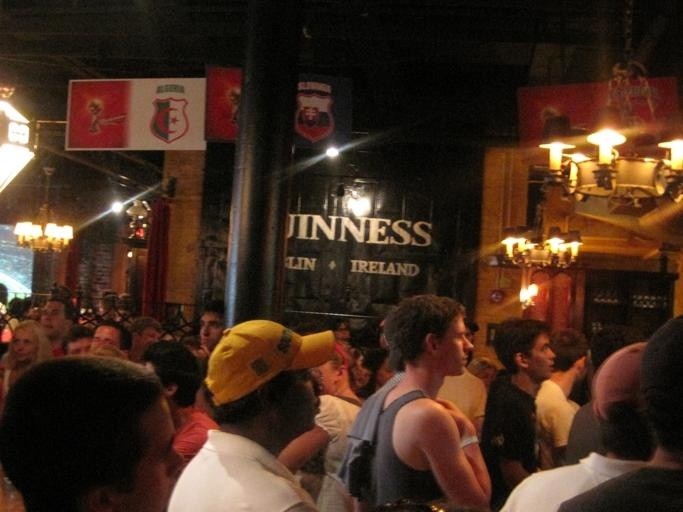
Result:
203 319 337 408
594 340 653 420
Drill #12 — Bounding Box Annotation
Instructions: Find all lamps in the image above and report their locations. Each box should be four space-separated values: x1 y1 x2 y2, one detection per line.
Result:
13 157 74 255
500 204 584 275
126 199 147 220
539 1 683 208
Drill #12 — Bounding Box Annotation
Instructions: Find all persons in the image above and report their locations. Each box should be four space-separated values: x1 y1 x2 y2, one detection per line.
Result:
1 273 683 512
